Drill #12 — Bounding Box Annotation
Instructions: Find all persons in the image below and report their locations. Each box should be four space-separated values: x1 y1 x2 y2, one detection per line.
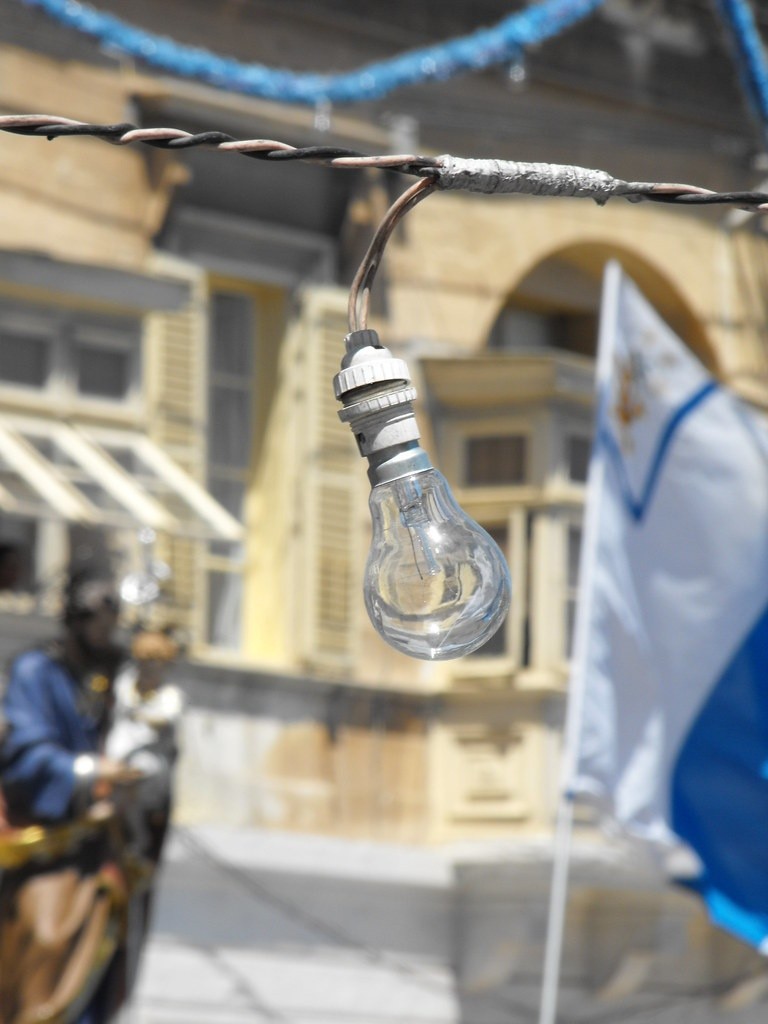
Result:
0 565 180 1024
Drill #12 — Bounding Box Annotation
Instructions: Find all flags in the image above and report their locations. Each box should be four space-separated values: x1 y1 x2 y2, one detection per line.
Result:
564 275 768 959
